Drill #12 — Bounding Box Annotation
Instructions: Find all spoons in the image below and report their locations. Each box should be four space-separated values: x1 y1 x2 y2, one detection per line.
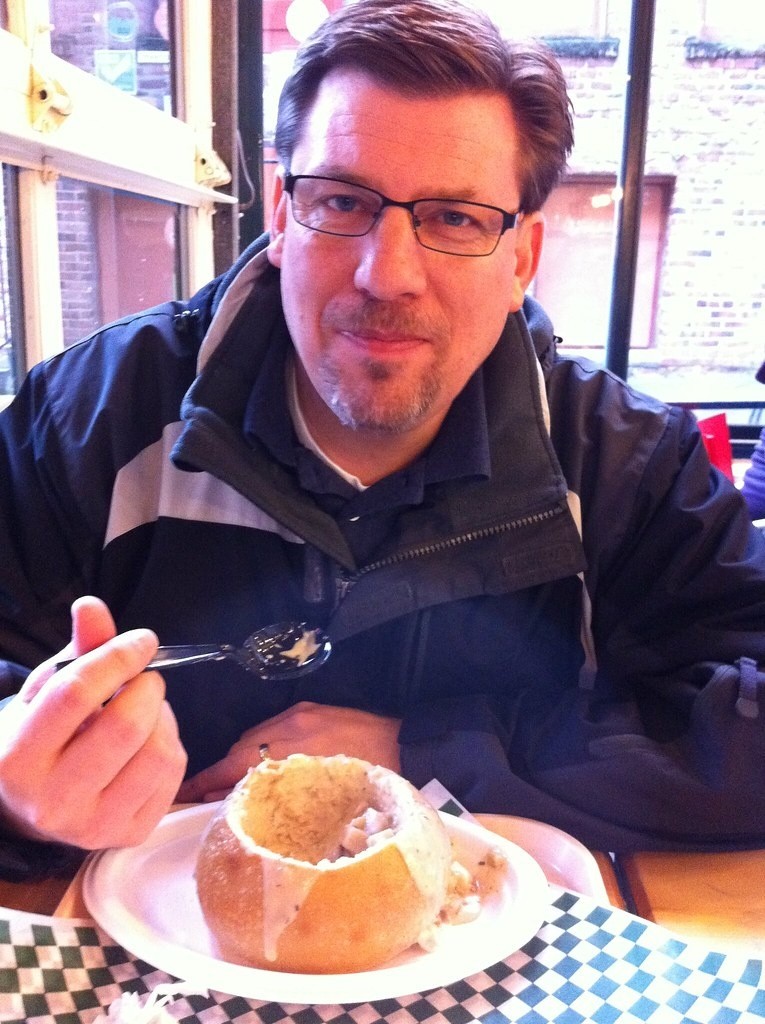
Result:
57 623 332 680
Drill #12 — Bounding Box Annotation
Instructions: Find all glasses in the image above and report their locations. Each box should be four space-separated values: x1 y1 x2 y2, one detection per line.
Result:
282 169 527 257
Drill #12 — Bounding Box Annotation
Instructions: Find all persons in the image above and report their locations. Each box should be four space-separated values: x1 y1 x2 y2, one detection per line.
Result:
0 0 765 885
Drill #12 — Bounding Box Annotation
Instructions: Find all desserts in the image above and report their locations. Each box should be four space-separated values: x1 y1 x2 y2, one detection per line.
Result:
195 754 451 972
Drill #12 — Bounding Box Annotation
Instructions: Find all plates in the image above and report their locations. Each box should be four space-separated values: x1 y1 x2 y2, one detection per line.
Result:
81 798 549 1004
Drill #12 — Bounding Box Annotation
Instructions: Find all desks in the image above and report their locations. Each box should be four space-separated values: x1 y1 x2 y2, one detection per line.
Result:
0 803 625 1024
611 851 765 1024
628 374 765 410
